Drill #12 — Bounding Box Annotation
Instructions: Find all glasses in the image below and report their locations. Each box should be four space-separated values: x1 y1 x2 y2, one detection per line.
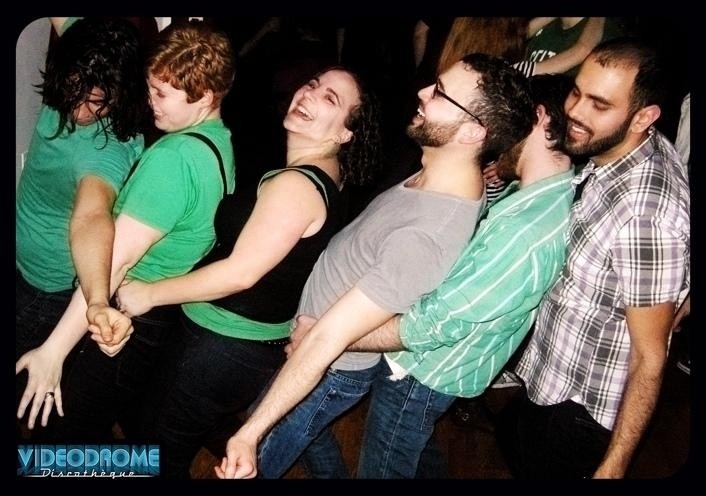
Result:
433 83 483 127
